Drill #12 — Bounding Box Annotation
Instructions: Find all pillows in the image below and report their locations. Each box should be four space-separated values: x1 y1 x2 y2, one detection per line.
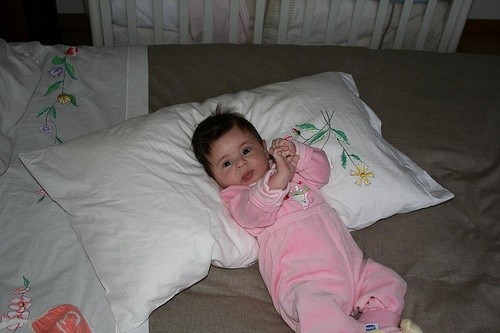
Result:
17 71 455 333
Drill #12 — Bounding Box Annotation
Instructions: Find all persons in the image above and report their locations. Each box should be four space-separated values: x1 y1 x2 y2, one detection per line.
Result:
191 112 408 333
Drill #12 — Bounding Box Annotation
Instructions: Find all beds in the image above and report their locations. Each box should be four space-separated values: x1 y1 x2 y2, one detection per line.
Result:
0 40 500 333
